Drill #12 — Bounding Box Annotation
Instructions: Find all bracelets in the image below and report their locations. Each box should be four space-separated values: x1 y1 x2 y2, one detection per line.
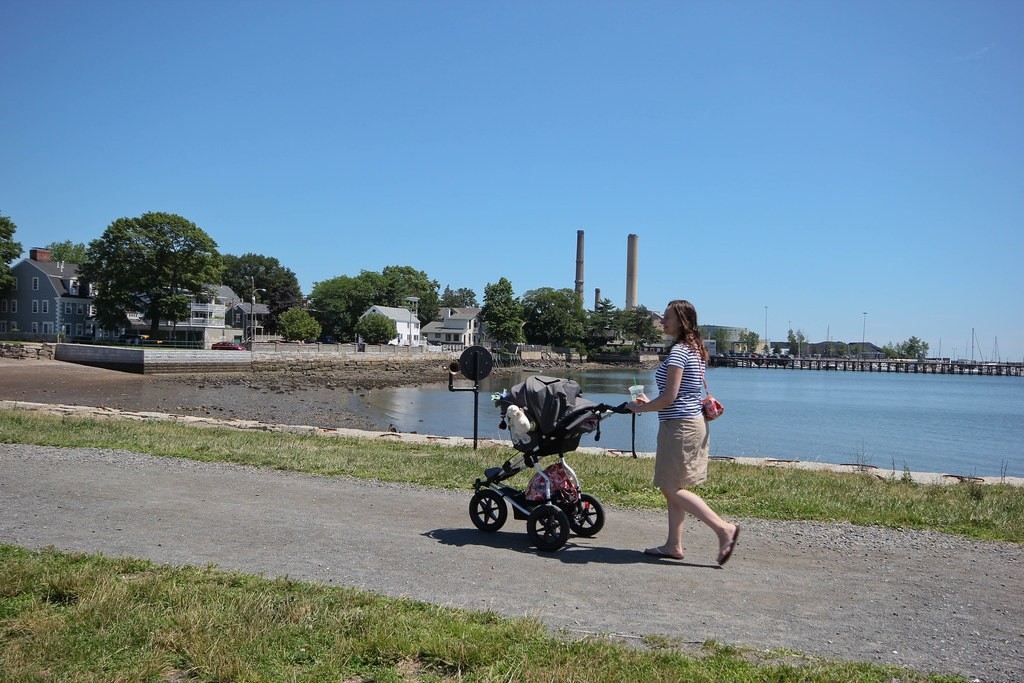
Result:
636 404 641 416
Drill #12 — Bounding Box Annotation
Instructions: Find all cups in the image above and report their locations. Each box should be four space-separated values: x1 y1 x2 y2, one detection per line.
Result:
629 385 644 402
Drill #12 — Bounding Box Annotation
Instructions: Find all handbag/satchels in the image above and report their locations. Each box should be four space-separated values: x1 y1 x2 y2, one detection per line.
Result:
525 463 578 503
702 395 725 421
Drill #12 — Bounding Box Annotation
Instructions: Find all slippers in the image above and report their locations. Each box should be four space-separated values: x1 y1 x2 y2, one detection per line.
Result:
717 523 740 565
643 546 685 561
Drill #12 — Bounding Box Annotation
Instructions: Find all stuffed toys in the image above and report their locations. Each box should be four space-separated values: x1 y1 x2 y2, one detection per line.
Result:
507 405 531 435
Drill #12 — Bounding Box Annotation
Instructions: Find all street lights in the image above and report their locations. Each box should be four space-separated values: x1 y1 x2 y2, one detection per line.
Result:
861 311 868 359
249 287 268 363
764 306 768 343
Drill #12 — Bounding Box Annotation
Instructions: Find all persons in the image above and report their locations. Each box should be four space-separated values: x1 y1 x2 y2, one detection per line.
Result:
626 300 741 565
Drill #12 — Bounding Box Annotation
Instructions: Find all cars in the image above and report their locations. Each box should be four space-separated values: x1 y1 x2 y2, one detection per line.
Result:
211 341 247 350
119 334 142 345
267 340 282 344
319 336 335 344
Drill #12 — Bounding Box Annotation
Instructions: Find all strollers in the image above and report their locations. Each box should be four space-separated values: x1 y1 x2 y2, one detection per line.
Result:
469 375 637 553
